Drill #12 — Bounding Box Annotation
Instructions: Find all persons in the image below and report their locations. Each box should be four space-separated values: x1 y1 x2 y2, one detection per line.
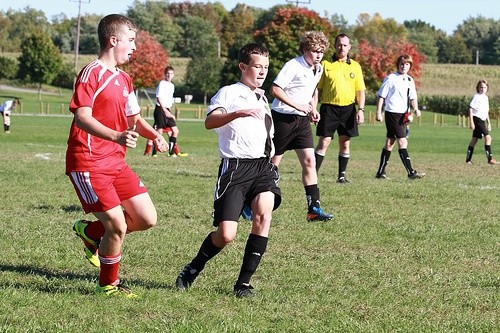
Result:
374 54 427 180
0 99 17 134
314 33 367 185
242 31 334 222
175 42 282 300
65 14 169 301
465 80 500 166
144 66 189 158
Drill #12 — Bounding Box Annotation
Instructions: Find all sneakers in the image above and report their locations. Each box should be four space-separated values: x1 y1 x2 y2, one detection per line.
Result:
376 174 390 180
94 284 138 298
175 263 201 289
243 206 253 221
234 284 254 300
408 171 425 179
307 208 334 222
73 220 101 267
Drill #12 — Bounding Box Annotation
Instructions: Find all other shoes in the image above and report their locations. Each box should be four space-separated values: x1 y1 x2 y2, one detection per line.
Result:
168 153 178 157
143 153 151 157
488 159 499 165
336 177 350 183
178 152 188 157
466 162 473 166
151 153 158 158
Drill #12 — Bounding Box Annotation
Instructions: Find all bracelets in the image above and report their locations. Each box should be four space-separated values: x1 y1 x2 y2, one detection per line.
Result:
358 108 364 113
